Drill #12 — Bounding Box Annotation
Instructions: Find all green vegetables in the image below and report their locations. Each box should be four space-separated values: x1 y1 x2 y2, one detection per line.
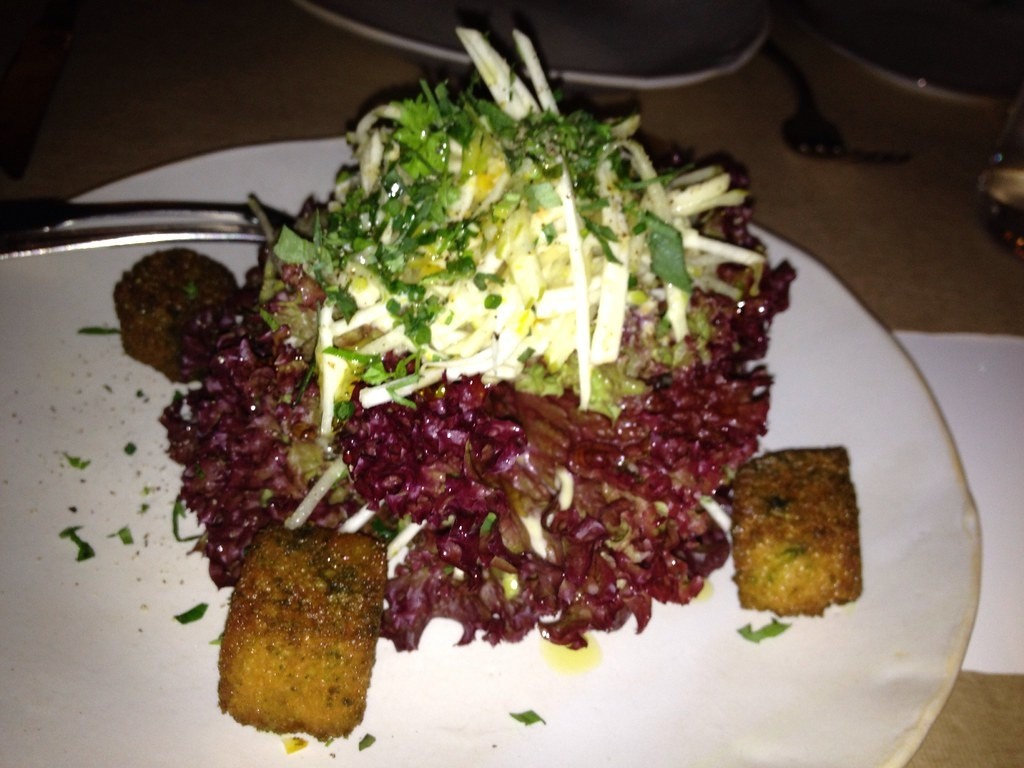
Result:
58 74 699 727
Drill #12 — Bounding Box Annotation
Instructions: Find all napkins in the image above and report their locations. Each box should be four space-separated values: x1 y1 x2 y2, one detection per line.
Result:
872 329 1024 679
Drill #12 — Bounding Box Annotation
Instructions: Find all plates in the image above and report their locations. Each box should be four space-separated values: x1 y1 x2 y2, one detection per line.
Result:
299 1 773 94
0 129 978 768
788 0 1024 110
